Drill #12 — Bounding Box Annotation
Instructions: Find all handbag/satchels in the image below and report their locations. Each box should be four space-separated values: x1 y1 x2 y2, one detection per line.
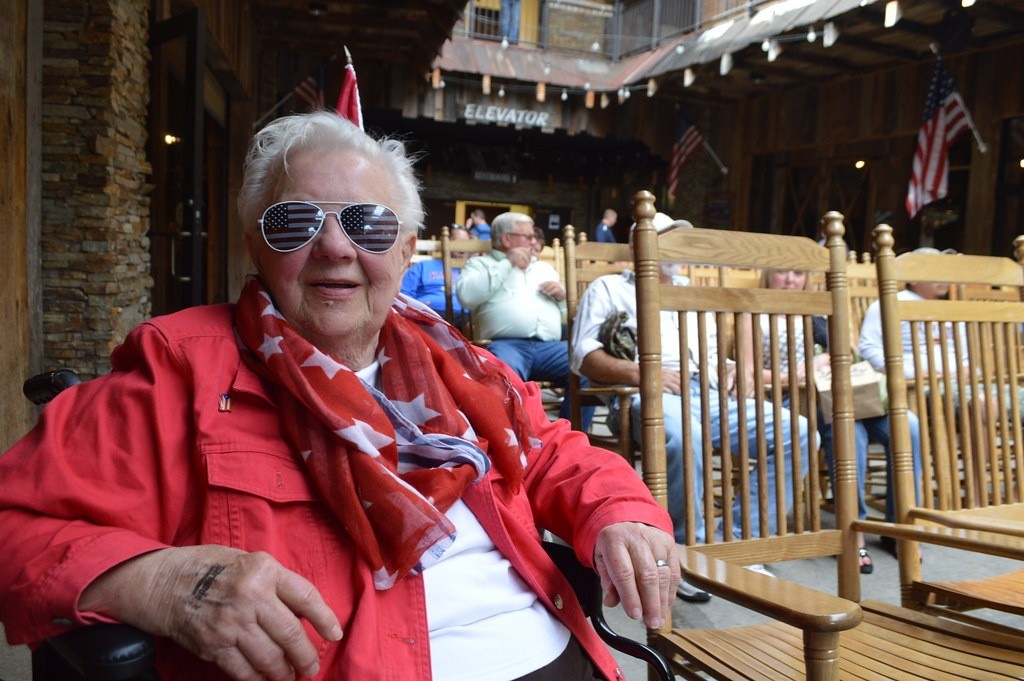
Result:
814 361 886 424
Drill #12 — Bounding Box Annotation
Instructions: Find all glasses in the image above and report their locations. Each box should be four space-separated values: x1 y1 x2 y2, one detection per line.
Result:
506 231 538 240
257 200 408 253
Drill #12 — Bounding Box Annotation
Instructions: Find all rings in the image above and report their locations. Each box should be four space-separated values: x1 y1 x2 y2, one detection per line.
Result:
656 560 669 568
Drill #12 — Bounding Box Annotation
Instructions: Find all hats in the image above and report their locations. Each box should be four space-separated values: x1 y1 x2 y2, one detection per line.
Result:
910 248 956 254
629 211 694 245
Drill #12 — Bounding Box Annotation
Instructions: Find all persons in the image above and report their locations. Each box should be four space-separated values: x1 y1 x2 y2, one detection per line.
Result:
0 108 680 681
401 209 1024 603
500 0 521 45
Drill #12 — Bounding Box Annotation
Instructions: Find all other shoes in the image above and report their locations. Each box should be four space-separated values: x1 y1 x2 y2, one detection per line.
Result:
678 578 712 601
859 547 873 574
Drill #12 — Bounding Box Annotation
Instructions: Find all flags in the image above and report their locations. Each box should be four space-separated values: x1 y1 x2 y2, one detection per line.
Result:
340 204 398 253
263 202 319 250
335 67 364 133
664 105 706 203
904 54 976 219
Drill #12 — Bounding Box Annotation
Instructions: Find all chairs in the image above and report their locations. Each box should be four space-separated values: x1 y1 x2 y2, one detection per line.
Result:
411 190 1023 681
